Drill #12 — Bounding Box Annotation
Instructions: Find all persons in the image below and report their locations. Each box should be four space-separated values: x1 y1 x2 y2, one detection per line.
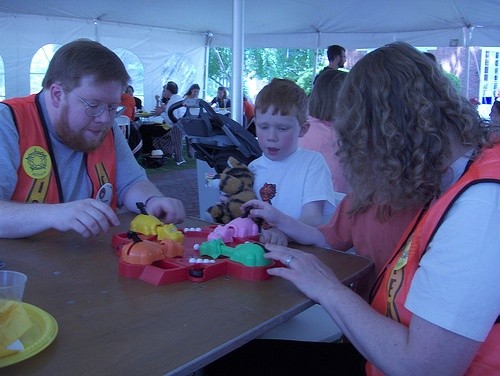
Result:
124 85 142 121
243 94 257 137
138 82 187 166
183 84 200 100
0 39 188 238
307 44 346 95
218 78 337 243
210 87 231 118
155 85 170 109
199 41 500 376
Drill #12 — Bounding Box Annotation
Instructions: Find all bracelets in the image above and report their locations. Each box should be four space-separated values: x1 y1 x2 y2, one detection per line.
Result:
144 195 163 207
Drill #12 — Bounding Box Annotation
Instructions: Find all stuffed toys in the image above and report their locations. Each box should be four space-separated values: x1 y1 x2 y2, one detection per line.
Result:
206 156 263 229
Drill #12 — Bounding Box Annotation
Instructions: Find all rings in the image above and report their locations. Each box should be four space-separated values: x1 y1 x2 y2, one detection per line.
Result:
287 255 296 268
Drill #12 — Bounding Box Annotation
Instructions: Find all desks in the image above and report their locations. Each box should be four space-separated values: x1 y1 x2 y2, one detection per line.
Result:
0 212 374 376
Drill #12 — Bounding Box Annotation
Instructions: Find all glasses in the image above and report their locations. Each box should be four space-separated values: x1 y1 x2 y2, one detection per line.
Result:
71 91 128 119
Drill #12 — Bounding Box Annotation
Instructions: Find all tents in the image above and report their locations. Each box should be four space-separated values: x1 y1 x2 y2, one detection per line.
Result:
0 0 499 106
